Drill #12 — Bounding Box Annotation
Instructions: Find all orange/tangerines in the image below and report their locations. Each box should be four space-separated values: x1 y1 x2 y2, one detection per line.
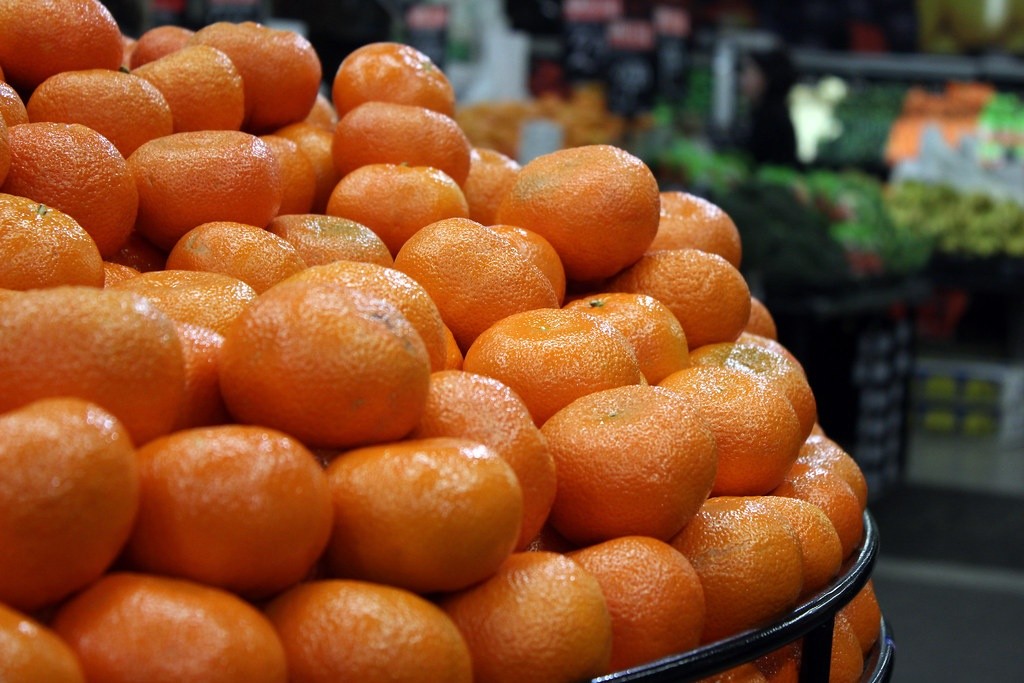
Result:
0 0 879 683
